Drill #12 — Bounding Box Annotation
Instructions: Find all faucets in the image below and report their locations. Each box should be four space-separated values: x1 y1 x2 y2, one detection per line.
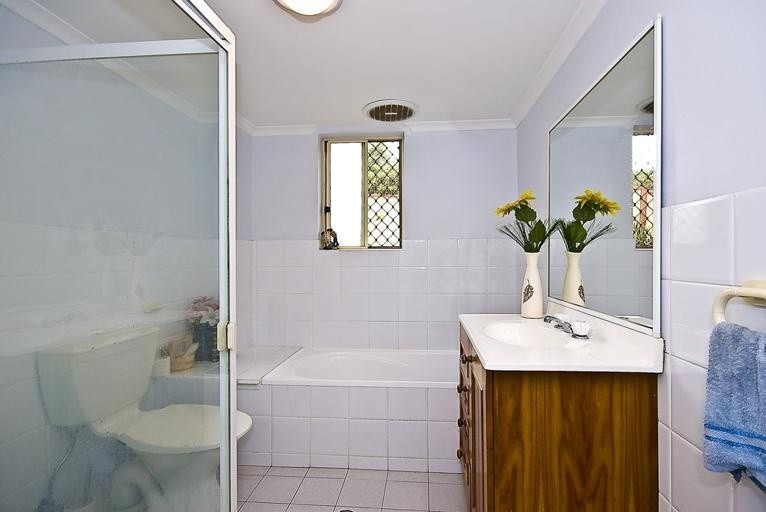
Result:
544 316 574 335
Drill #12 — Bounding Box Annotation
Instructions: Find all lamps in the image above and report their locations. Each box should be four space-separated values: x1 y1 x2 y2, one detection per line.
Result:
276 1 346 17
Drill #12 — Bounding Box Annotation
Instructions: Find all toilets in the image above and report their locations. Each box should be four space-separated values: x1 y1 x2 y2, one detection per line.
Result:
36 325 253 512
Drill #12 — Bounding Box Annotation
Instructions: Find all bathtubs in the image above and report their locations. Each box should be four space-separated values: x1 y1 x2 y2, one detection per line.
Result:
260 346 462 389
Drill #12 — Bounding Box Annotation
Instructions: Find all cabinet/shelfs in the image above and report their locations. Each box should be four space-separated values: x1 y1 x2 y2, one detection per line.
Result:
457 323 660 512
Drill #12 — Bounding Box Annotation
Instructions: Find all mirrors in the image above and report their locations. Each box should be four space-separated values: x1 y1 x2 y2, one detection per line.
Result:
547 13 661 338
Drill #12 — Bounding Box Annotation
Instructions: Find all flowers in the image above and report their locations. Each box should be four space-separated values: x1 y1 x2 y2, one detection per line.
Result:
184 296 219 326
493 190 568 254
553 188 622 253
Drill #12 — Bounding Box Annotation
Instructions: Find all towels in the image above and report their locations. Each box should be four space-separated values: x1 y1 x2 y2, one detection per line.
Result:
705 322 765 491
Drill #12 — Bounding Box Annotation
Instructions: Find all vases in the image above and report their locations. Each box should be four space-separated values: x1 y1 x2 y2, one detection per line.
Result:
562 252 585 306
193 322 220 361
521 251 543 317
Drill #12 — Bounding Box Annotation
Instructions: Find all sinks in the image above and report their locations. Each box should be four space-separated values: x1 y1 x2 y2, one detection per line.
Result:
480 322 570 352
